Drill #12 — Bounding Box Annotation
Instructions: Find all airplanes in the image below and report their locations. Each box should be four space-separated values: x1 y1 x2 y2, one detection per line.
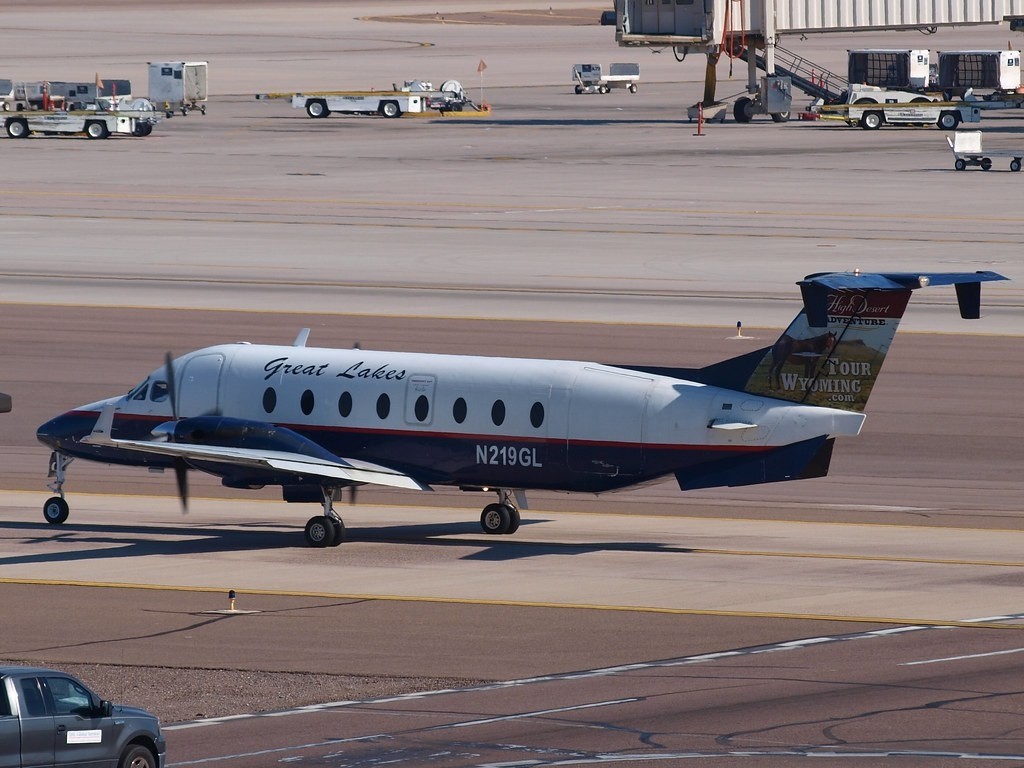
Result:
35 270 1012 549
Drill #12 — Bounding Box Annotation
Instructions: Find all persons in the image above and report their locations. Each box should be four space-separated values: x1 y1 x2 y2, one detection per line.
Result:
799 33 808 42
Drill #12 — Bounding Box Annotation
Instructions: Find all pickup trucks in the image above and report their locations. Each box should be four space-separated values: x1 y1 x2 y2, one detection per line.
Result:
0 664 167 768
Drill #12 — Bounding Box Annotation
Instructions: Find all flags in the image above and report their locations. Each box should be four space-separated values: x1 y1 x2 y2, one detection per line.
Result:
96 72 104 89
